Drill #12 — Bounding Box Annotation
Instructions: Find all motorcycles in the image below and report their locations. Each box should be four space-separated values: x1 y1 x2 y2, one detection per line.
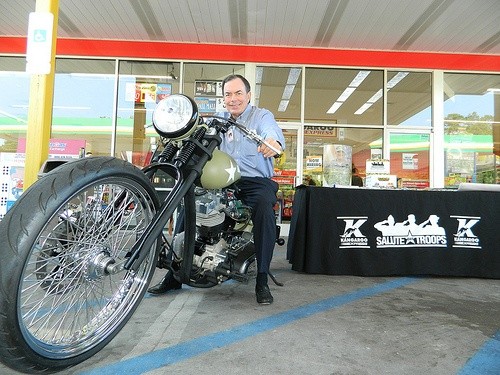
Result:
0 94 285 375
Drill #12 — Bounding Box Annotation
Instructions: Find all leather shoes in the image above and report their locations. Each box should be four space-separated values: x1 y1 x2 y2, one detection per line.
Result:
255 284 273 305
147 277 182 294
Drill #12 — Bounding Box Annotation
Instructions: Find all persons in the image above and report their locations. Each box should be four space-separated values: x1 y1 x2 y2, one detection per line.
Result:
351 168 363 187
306 176 316 186
147 74 286 305
323 145 351 188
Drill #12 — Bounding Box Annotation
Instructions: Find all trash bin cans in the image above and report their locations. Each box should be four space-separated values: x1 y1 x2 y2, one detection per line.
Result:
38 157 77 247
